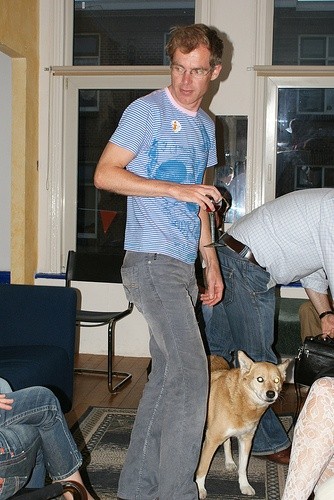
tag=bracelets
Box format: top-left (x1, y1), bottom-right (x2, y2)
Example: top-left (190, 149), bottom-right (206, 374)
top-left (319, 311), bottom-right (334, 319)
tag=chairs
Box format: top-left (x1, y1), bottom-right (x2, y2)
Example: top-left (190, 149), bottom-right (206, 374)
top-left (64, 251), bottom-right (134, 394)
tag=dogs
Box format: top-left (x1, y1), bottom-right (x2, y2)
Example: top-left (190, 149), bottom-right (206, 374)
top-left (194, 350), bottom-right (292, 500)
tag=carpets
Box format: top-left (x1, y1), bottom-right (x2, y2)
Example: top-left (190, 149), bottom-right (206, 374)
top-left (44, 406), bottom-right (295, 500)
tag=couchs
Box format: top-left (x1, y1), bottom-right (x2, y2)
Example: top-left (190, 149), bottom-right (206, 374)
top-left (0, 280), bottom-right (82, 416)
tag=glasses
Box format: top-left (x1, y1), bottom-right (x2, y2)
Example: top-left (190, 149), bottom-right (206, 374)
top-left (170, 62), bottom-right (214, 79)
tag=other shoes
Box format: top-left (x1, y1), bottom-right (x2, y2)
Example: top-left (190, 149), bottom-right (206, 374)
top-left (253, 445), bottom-right (291, 465)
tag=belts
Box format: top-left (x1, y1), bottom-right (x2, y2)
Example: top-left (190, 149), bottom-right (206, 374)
top-left (218, 229), bottom-right (267, 271)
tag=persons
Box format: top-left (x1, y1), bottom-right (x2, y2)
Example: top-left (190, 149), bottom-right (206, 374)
top-left (0, 386), bottom-right (95, 500)
top-left (299, 288), bottom-right (334, 345)
top-left (211, 188), bottom-right (334, 466)
top-left (282, 377), bottom-right (334, 500)
top-left (94, 23), bottom-right (224, 500)
top-left (214, 165), bottom-right (235, 223)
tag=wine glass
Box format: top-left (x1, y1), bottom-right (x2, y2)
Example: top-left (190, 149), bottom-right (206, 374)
top-left (202, 196), bottom-right (227, 248)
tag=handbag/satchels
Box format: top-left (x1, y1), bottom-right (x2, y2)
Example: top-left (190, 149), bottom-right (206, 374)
top-left (293, 333), bottom-right (334, 388)
